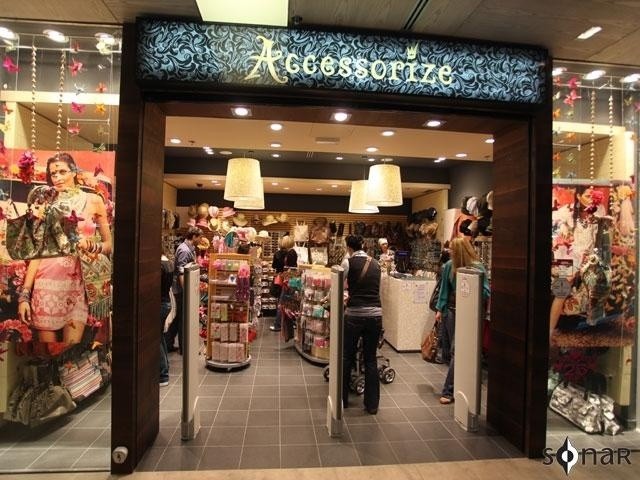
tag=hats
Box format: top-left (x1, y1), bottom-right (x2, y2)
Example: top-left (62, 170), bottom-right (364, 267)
top-left (460, 190), bottom-right (493, 234)
top-left (378, 238), bottom-right (388, 246)
top-left (187, 203), bottom-right (221, 232)
top-left (255, 230), bottom-right (271, 242)
top-left (406, 208), bottom-right (438, 240)
top-left (223, 206), bottom-right (289, 227)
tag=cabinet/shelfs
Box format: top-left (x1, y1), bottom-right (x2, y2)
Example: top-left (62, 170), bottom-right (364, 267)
top-left (205, 255), bottom-right (252, 369)
top-left (293, 265), bottom-right (331, 364)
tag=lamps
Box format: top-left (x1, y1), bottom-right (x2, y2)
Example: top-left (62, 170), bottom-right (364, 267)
top-left (223, 153), bottom-right (261, 201)
top-left (366, 158), bottom-right (404, 207)
top-left (348, 166), bottom-right (380, 214)
top-left (234, 178), bottom-right (265, 210)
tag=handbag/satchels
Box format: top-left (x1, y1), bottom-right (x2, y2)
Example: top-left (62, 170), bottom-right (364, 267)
top-left (3, 350), bottom-right (104, 429)
top-left (6, 185), bottom-right (79, 259)
top-left (293, 225), bottom-right (309, 242)
top-left (311, 247), bottom-right (328, 266)
top-left (311, 226), bottom-right (332, 244)
top-left (421, 321), bottom-right (444, 364)
top-left (429, 279), bottom-right (441, 312)
top-left (293, 247), bottom-right (308, 264)
top-left (77, 236), bottom-right (113, 319)
top-left (547, 367), bottom-right (620, 436)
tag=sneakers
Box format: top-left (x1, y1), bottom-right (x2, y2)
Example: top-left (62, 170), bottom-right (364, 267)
top-left (159, 381), bottom-right (169, 386)
top-left (167, 347), bottom-right (179, 352)
top-left (270, 326), bottom-right (281, 331)
top-left (440, 397), bottom-right (451, 404)
top-left (364, 403), bottom-right (378, 414)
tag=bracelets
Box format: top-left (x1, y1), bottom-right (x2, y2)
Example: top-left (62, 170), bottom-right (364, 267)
top-left (85, 238), bottom-right (102, 255)
top-left (17, 290), bottom-right (31, 304)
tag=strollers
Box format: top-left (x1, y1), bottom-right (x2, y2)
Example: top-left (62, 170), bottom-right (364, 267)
top-left (321, 326), bottom-right (399, 402)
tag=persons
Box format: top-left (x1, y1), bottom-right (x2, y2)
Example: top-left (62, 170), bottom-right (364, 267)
top-left (550, 185), bottom-right (607, 332)
top-left (159, 248), bottom-right (175, 387)
top-left (377, 237), bottom-right (396, 273)
top-left (17, 152), bottom-right (113, 345)
top-left (268, 235), bottom-right (298, 332)
top-left (165, 225), bottom-right (204, 354)
top-left (434, 236), bottom-right (490, 404)
top-left (340, 235), bottom-right (384, 414)
